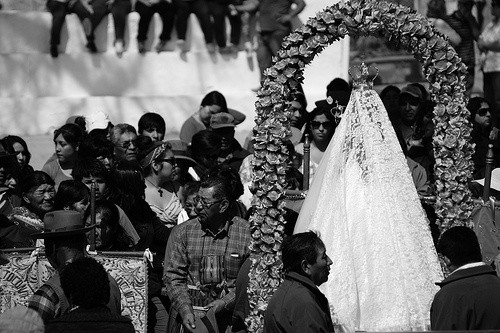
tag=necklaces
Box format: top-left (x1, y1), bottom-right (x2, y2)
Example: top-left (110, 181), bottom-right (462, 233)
top-left (147, 179), bottom-right (163, 197)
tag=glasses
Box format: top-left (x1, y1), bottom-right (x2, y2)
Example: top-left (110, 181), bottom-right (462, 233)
top-left (117, 140), bottom-right (135, 147)
top-left (156, 157), bottom-right (175, 165)
top-left (476, 107), bottom-right (493, 116)
top-left (193, 196), bottom-right (225, 207)
top-left (310, 121), bottom-right (331, 129)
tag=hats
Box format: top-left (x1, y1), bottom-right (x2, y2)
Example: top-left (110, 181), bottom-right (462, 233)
top-left (0, 139), bottom-right (20, 156)
top-left (400, 86), bottom-right (422, 97)
top-left (86, 112), bottom-right (111, 134)
top-left (164, 139), bottom-right (198, 167)
top-left (30, 210), bottom-right (99, 237)
top-left (211, 111), bottom-right (236, 129)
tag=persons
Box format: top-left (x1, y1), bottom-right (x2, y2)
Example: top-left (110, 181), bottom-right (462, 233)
top-left (0, 75), bottom-right (500, 333)
top-left (45, 1), bottom-right (307, 91)
top-left (414, 0), bottom-right (500, 128)
